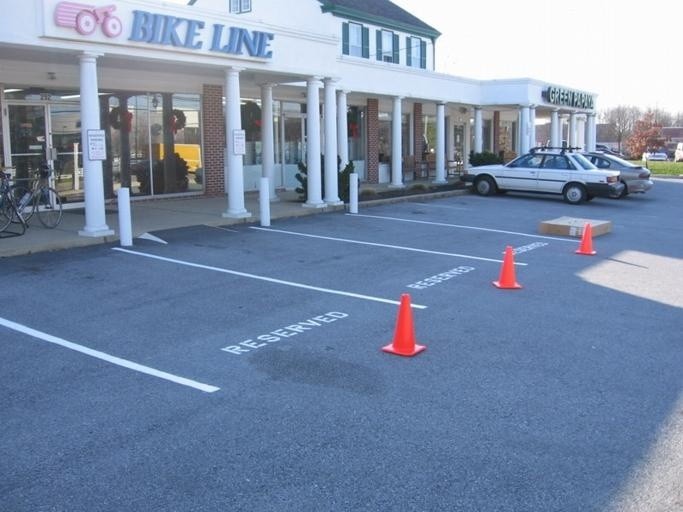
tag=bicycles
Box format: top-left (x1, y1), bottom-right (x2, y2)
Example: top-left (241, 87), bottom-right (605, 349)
top-left (0, 165), bottom-right (62, 233)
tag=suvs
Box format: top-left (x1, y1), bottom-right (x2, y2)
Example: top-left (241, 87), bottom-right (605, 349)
top-left (675, 142), bottom-right (682, 161)
top-left (595, 143), bottom-right (624, 158)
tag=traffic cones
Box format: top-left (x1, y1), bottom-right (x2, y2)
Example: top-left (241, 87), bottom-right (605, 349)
top-left (577, 225), bottom-right (596, 255)
top-left (383, 294), bottom-right (425, 357)
top-left (495, 246), bottom-right (522, 289)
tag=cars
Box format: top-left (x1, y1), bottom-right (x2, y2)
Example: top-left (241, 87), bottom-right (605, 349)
top-left (642, 152), bottom-right (668, 161)
top-left (581, 152), bottom-right (653, 199)
top-left (460, 151), bottom-right (620, 205)
